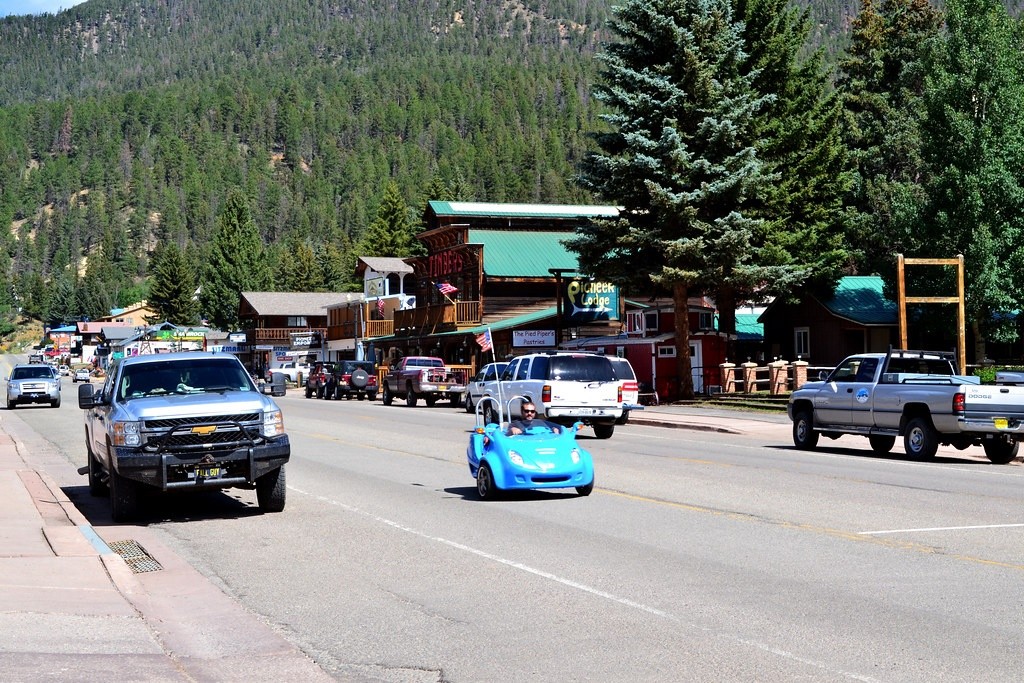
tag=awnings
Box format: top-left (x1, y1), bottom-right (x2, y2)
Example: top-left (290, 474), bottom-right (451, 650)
top-left (115, 333), bottom-right (145, 346)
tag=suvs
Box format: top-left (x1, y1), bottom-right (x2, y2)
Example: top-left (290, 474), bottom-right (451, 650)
top-left (305, 363), bottom-right (336, 399)
top-left (324, 360), bottom-right (379, 401)
top-left (3, 364), bottom-right (61, 409)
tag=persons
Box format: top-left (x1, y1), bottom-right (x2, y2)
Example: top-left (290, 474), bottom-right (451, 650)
top-left (508, 402), bottom-right (559, 436)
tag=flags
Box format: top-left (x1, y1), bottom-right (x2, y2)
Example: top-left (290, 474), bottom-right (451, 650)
top-left (476, 330), bottom-right (492, 352)
top-left (434, 283), bottom-right (457, 295)
top-left (376, 296), bottom-right (384, 312)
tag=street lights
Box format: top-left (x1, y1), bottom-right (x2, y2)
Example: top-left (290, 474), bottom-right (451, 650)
top-left (176, 328), bottom-right (188, 352)
top-left (345, 292), bottom-right (366, 361)
top-left (56, 333), bottom-right (61, 367)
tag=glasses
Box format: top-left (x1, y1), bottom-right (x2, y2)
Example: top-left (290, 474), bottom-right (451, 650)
top-left (523, 409), bottom-right (535, 413)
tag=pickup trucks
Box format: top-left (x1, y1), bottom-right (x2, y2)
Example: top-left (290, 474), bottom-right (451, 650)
top-left (383, 356), bottom-right (466, 408)
top-left (72, 368), bottom-right (90, 384)
top-left (27, 354), bottom-right (41, 365)
top-left (268, 362), bottom-right (313, 382)
top-left (786, 344), bottom-right (1024, 464)
top-left (77, 352), bottom-right (292, 522)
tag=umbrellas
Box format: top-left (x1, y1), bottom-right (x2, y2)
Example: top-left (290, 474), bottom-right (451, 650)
top-left (355, 340), bottom-right (365, 361)
top-left (366, 340), bottom-right (376, 365)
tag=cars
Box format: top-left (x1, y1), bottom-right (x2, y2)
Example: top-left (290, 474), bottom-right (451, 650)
top-left (47, 362), bottom-right (60, 374)
top-left (59, 365), bottom-right (69, 376)
top-left (481, 350), bottom-right (623, 439)
top-left (602, 354), bottom-right (645, 426)
top-left (466, 419), bottom-right (596, 500)
top-left (465, 362), bottom-right (511, 413)
